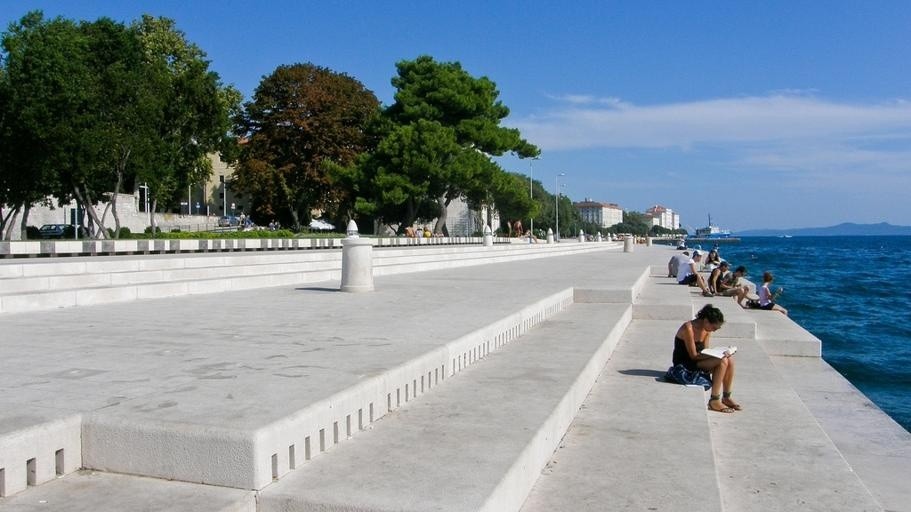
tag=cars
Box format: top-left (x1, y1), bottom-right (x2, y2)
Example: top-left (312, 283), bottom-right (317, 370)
top-left (216, 214), bottom-right (240, 226)
top-left (37, 223), bottom-right (70, 238)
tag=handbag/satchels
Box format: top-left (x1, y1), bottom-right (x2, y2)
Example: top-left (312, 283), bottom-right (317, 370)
top-left (666, 364), bottom-right (713, 391)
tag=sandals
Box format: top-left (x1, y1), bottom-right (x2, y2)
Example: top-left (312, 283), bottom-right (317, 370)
top-left (708, 392), bottom-right (743, 413)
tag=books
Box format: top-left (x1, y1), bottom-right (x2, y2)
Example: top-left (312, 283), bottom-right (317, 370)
top-left (703, 346), bottom-right (737, 359)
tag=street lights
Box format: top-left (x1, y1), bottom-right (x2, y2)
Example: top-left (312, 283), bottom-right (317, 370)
top-left (231, 202), bottom-right (236, 217)
top-left (219, 173), bottom-right (227, 215)
top-left (529, 156), bottom-right (542, 243)
top-left (187, 182), bottom-right (197, 215)
top-left (559, 182), bottom-right (567, 194)
top-left (554, 171), bottom-right (566, 241)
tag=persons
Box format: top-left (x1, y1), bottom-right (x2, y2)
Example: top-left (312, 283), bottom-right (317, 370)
top-left (423, 227), bottom-right (432, 237)
top-left (514, 220), bottom-right (523, 238)
top-left (667, 248), bottom-right (787, 316)
top-left (268, 218), bottom-right (275, 231)
top-left (240, 211), bottom-right (245, 225)
top-left (245, 215), bottom-right (254, 227)
top-left (275, 220), bottom-right (281, 230)
top-left (506, 218), bottom-right (512, 237)
top-left (415, 227), bottom-right (423, 238)
top-left (672, 303), bottom-right (743, 413)
top-left (404, 225), bottom-right (415, 238)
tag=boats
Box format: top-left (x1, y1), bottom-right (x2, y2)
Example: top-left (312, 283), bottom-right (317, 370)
top-left (688, 211), bottom-right (736, 238)
top-left (781, 234), bottom-right (792, 238)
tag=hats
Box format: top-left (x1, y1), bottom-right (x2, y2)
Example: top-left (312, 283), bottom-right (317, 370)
top-left (693, 250), bottom-right (704, 256)
